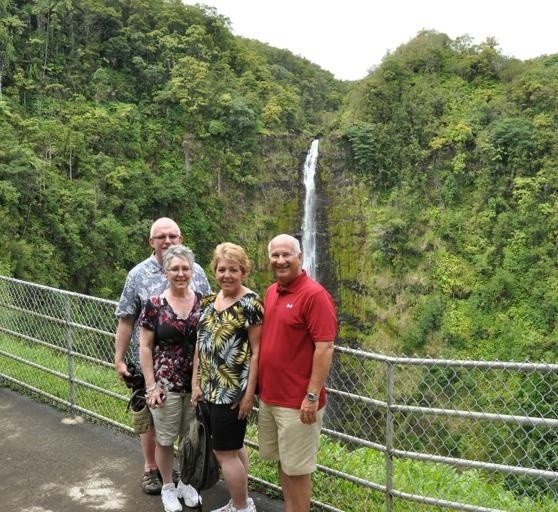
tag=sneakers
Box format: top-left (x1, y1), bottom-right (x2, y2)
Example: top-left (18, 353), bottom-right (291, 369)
top-left (142, 467), bottom-right (182, 495)
top-left (210, 498), bottom-right (257, 512)
top-left (176, 478), bottom-right (202, 508)
top-left (161, 482), bottom-right (182, 512)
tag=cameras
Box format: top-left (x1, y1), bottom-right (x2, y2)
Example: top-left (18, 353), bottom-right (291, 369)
top-left (123, 363), bottom-right (146, 389)
top-left (155, 394), bottom-right (167, 408)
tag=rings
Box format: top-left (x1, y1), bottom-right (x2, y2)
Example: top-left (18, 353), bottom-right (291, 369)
top-left (190, 399), bottom-right (192, 402)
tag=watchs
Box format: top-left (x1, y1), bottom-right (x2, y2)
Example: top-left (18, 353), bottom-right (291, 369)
top-left (306, 392), bottom-right (320, 401)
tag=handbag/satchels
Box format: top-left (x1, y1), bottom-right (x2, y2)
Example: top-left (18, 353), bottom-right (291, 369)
top-left (178, 415), bottom-right (219, 490)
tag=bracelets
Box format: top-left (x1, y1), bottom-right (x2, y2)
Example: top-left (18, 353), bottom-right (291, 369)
top-left (146, 383), bottom-right (157, 392)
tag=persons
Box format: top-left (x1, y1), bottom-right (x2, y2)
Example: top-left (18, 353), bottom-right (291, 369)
top-left (257, 233), bottom-right (337, 512)
top-left (191, 242), bottom-right (264, 512)
top-left (113, 217), bottom-right (211, 495)
top-left (137, 244), bottom-right (213, 512)
